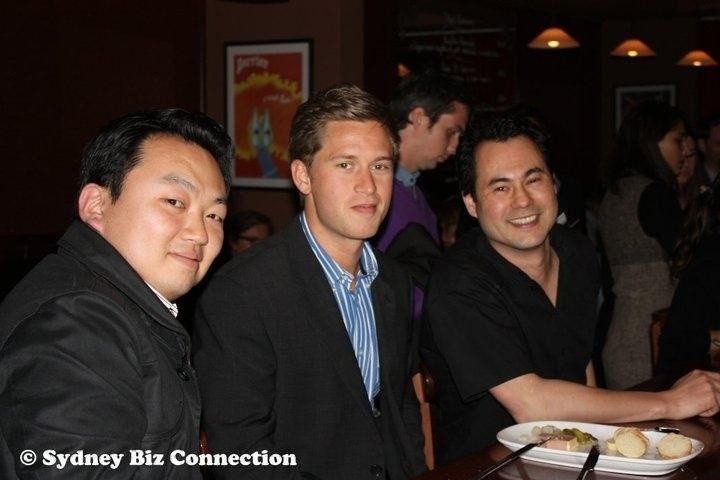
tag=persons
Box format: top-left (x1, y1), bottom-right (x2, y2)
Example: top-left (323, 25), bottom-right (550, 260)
top-left (419, 118), bottom-right (720, 479)
top-left (225, 209), bottom-right (274, 261)
top-left (695, 118), bottom-right (720, 199)
top-left (596, 110), bottom-right (720, 387)
top-left (187, 85), bottom-right (429, 480)
top-left (662, 132), bottom-right (699, 204)
top-left (0, 107), bottom-right (236, 480)
top-left (374, 79), bottom-right (470, 321)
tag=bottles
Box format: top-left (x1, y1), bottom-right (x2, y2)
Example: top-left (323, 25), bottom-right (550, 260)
top-left (261, 110), bottom-right (271, 149)
top-left (251, 108), bottom-right (261, 149)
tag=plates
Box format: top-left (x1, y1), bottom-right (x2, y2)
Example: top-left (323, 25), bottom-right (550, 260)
top-left (497, 421), bottom-right (704, 475)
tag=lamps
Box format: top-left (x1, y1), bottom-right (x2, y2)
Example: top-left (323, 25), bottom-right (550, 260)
top-left (528, 26), bottom-right (581, 50)
top-left (677, 48), bottom-right (717, 69)
top-left (611, 39), bottom-right (660, 59)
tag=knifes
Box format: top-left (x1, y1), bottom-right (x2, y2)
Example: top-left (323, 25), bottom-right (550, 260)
top-left (576, 443), bottom-right (602, 480)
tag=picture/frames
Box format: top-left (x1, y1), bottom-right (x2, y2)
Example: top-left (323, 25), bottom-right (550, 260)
top-left (225, 39), bottom-right (314, 193)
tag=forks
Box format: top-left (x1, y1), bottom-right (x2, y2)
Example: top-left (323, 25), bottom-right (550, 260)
top-left (477, 433), bottom-right (555, 480)
